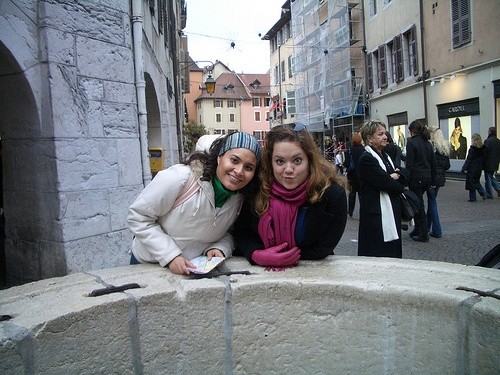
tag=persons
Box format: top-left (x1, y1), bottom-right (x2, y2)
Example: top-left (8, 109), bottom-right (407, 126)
top-left (191, 133), bottom-right (200, 144)
top-left (448, 117), bottom-right (467, 159)
top-left (127, 131), bottom-right (262, 277)
top-left (396, 126), bottom-right (406, 153)
top-left (316, 117), bottom-right (500, 257)
top-left (232, 123), bottom-right (347, 271)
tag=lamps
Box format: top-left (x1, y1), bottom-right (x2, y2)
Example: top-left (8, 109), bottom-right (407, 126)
top-left (178, 61), bottom-right (217, 97)
top-left (451, 73), bottom-right (467, 80)
top-left (429, 80), bottom-right (439, 86)
top-left (441, 77), bottom-right (451, 83)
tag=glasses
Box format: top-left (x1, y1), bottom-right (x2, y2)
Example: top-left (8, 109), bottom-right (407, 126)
top-left (270, 122), bottom-right (309, 133)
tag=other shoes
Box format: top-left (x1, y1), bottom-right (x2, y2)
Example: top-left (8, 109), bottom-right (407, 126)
top-left (482, 192), bottom-right (487, 200)
top-left (497, 188), bottom-right (500, 197)
top-left (428, 232), bottom-right (443, 237)
top-left (468, 199), bottom-right (477, 202)
top-left (412, 234), bottom-right (430, 242)
top-left (487, 196), bottom-right (493, 199)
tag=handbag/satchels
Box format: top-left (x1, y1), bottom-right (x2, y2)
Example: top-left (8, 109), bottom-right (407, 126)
top-left (397, 190), bottom-right (421, 221)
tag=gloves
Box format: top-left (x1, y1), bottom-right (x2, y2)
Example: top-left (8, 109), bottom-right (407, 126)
top-left (252, 242), bottom-right (301, 267)
